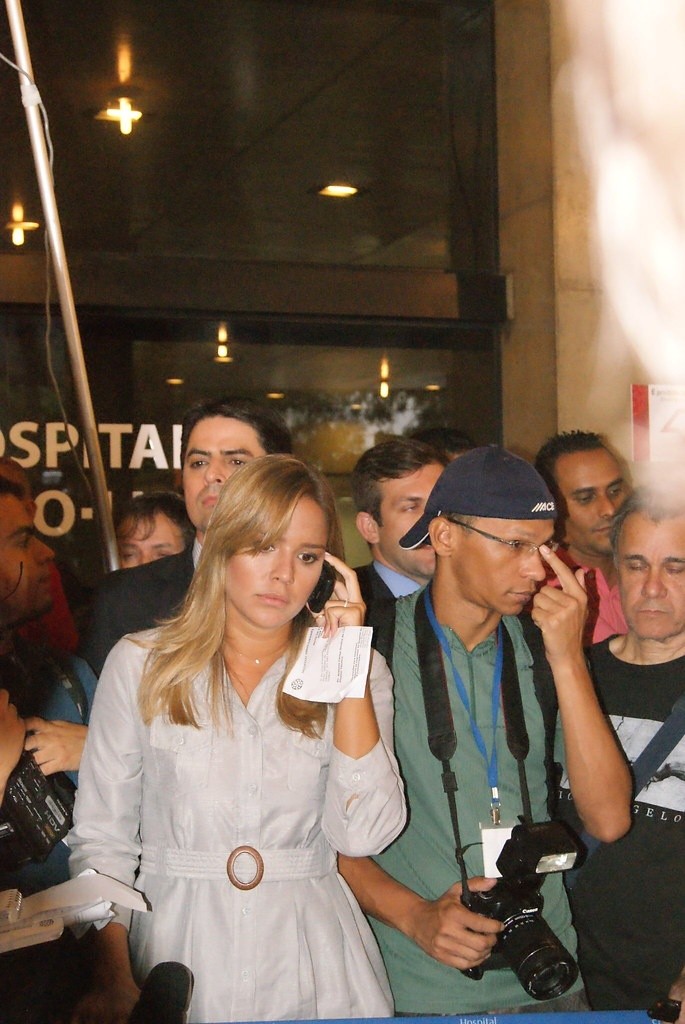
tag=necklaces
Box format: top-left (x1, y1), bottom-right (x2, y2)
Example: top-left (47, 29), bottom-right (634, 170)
top-left (232, 648), bottom-right (269, 664)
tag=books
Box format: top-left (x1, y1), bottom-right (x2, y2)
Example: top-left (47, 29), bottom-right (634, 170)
top-left (0, 889), bottom-right (22, 924)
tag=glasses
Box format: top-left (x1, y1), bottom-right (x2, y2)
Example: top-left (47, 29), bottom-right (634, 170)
top-left (444, 515), bottom-right (559, 563)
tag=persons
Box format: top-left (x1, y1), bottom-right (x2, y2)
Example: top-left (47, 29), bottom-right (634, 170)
top-left (412, 427), bottom-right (477, 462)
top-left (340, 438), bottom-right (454, 670)
top-left (546, 480), bottom-right (685, 1024)
top-left (87, 396), bottom-right (295, 677)
top-left (65, 454), bottom-right (410, 1024)
top-left (524, 431), bottom-right (630, 647)
top-left (111, 491), bottom-right (196, 570)
top-left (336, 444), bottom-right (634, 1013)
top-left (0, 458), bottom-right (97, 1024)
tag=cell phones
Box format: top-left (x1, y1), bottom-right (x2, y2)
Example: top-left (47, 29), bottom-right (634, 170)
top-left (307, 561), bottom-right (335, 612)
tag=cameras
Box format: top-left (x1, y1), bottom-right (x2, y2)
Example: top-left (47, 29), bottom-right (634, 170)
top-left (459, 821), bottom-right (587, 1000)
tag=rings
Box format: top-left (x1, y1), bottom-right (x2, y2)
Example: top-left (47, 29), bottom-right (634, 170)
top-left (344, 600), bottom-right (349, 608)
top-left (315, 614), bottom-right (325, 620)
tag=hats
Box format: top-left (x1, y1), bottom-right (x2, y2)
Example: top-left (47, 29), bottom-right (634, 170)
top-left (397, 443), bottom-right (559, 550)
